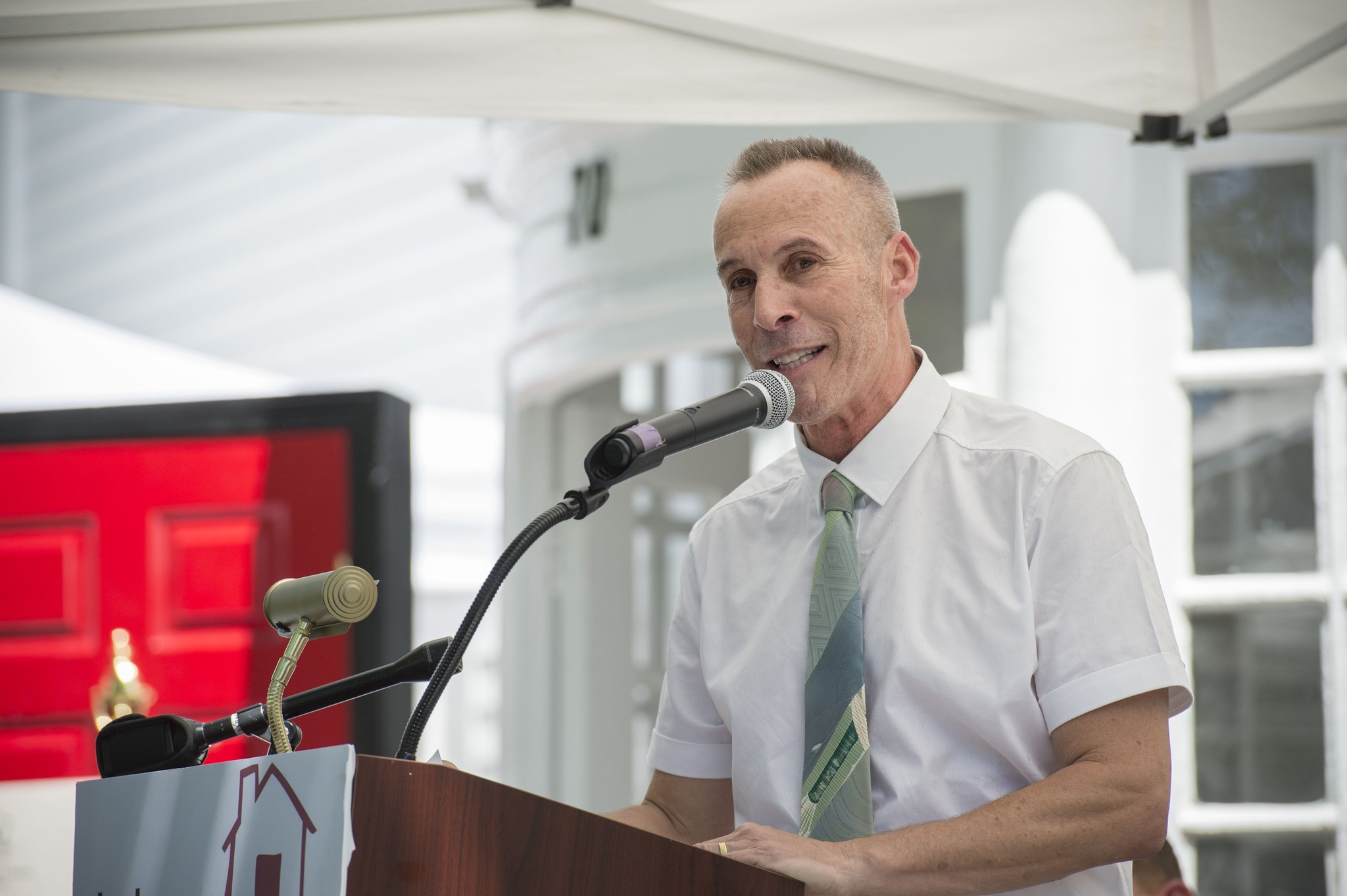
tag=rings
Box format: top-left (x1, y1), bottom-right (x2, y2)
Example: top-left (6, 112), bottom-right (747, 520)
top-left (716, 840), bottom-right (727, 856)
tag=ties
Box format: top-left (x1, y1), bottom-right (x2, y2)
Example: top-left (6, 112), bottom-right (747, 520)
top-left (799, 468), bottom-right (874, 844)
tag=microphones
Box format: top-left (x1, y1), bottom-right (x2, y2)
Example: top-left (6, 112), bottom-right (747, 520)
top-left (95, 637), bottom-right (464, 779)
top-left (603, 369), bottom-right (795, 473)
top-left (264, 566), bottom-right (378, 754)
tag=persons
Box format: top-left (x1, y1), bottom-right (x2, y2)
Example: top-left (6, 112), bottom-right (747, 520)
top-left (604, 135), bottom-right (1192, 896)
top-left (1133, 842), bottom-right (1198, 896)
